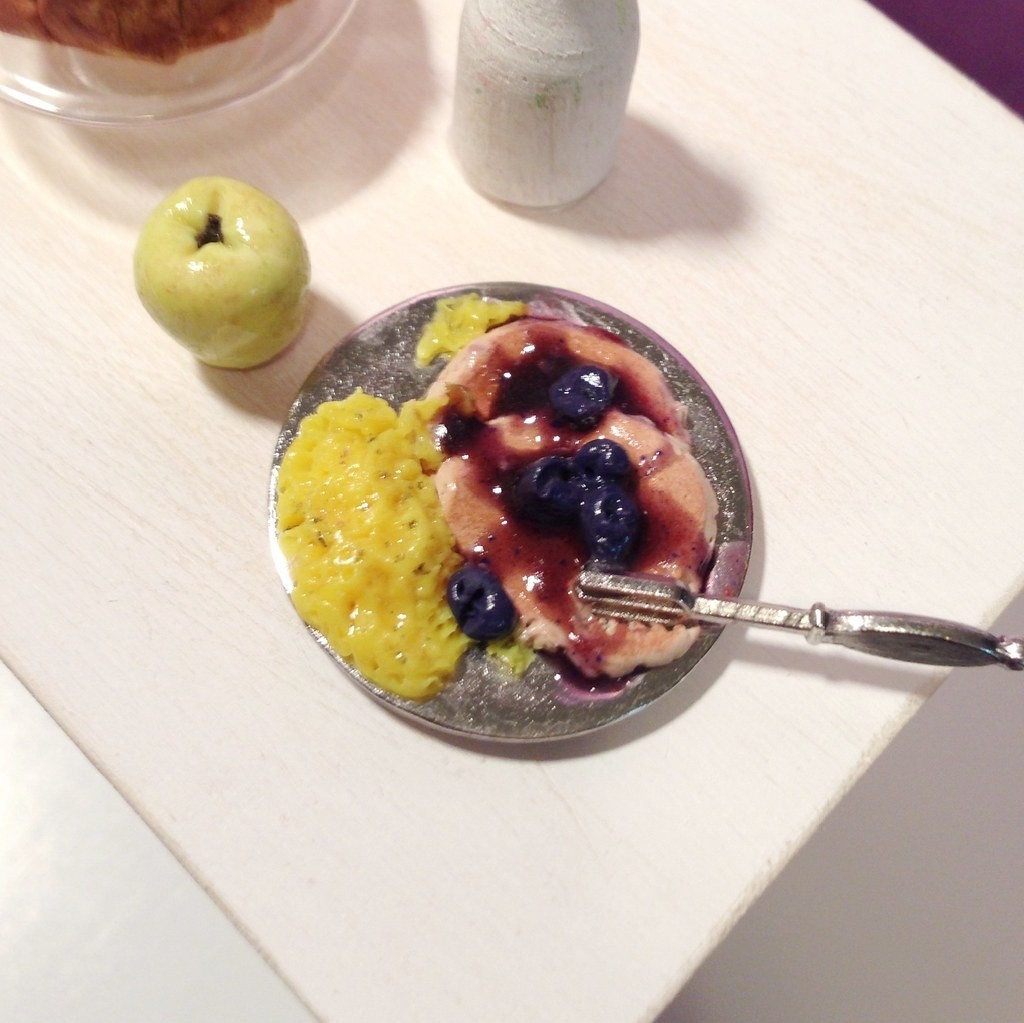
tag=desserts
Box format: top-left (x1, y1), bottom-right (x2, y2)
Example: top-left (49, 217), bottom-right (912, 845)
top-left (264, 282), bottom-right (755, 745)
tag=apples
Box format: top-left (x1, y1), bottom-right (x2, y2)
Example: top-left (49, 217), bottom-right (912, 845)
top-left (132, 175), bottom-right (310, 369)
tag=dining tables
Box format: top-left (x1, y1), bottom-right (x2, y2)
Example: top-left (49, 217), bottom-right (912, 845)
top-left (0, 0), bottom-right (1024, 1023)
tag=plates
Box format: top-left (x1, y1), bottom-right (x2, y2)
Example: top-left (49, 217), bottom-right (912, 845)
top-left (0, 0), bottom-right (355, 126)
top-left (267, 281), bottom-right (754, 744)
